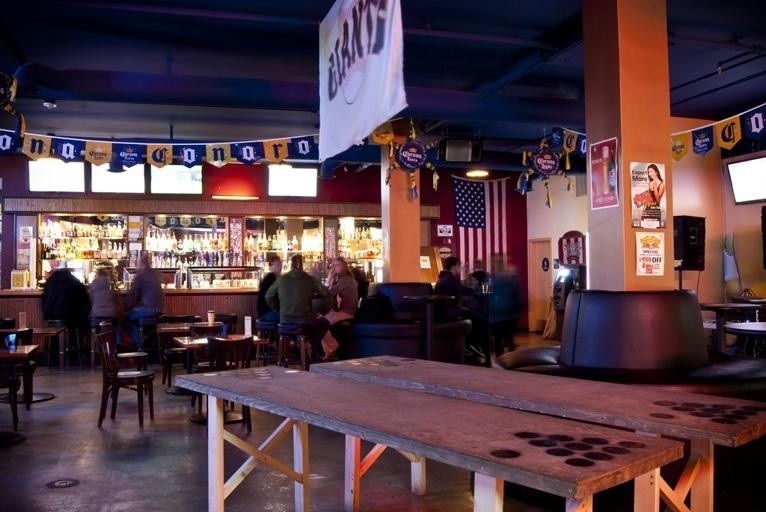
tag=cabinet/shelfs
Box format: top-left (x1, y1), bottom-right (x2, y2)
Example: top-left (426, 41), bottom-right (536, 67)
top-left (13, 210), bottom-right (386, 285)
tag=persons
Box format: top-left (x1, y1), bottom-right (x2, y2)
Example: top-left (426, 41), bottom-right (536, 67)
top-left (633, 164), bottom-right (665, 229)
top-left (257, 256), bottom-right (281, 321)
top-left (316, 257), bottom-right (359, 361)
top-left (88, 271), bottom-right (123, 353)
top-left (125, 253), bottom-right (162, 352)
top-left (432, 257), bottom-right (482, 321)
top-left (264, 256), bottom-right (332, 363)
top-left (42, 262), bottom-right (91, 335)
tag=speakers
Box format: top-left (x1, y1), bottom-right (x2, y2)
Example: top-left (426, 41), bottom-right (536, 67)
top-left (673, 216), bottom-right (706, 271)
top-left (439, 139), bottom-right (481, 163)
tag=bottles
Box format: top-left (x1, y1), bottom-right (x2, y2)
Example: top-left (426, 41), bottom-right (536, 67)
top-left (257, 233), bottom-right (262, 248)
top-left (171, 231), bottom-right (177, 250)
top-left (217, 248), bottom-right (223, 267)
top-left (182, 258), bottom-right (189, 287)
top-left (146, 226), bottom-right (171, 251)
top-left (244, 231), bottom-right (248, 250)
top-left (288, 239), bottom-right (292, 250)
top-left (189, 250), bottom-right (218, 266)
top-left (176, 257), bottom-right (183, 273)
top-left (47, 222), bottom-right (125, 238)
top-left (194, 234), bottom-right (199, 249)
top-left (165, 253), bottom-right (171, 269)
top-left (262, 233), bottom-right (267, 248)
top-left (129, 250), bottom-right (137, 269)
top-left (188, 234), bottom-right (193, 250)
top-left (244, 250), bottom-right (268, 269)
top-left (208, 233), bottom-right (213, 249)
top-left (183, 233), bottom-right (189, 250)
top-left (248, 232), bottom-right (255, 250)
top-left (267, 235), bottom-right (271, 249)
top-left (293, 235), bottom-right (298, 251)
top-left (153, 252), bottom-right (165, 269)
top-left (223, 248), bottom-right (229, 267)
top-left (199, 234), bottom-right (204, 250)
top-left (41, 241), bottom-right (128, 258)
top-left (302, 254), bottom-right (325, 274)
top-left (218, 234), bottom-right (223, 250)
top-left (172, 252), bottom-right (176, 269)
top-left (353, 225), bottom-right (371, 241)
top-left (272, 235), bottom-right (277, 249)
top-left (224, 233), bottom-right (229, 249)
top-left (204, 231), bottom-right (209, 249)
top-left (213, 232), bottom-right (218, 249)
top-left (229, 248), bottom-right (233, 266)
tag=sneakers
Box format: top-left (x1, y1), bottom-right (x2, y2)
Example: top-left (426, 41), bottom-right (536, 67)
top-left (466, 343), bottom-right (486, 356)
top-left (323, 343), bottom-right (339, 360)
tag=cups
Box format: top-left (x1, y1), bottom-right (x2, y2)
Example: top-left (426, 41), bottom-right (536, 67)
top-left (208, 310), bottom-right (215, 326)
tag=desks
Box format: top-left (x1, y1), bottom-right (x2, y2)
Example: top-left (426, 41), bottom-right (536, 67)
top-left (174, 333), bottom-right (252, 396)
top-left (403, 293), bottom-right (455, 362)
top-left (698, 303), bottom-right (761, 359)
top-left (313, 354), bottom-right (766, 512)
top-left (2, 344), bottom-right (39, 430)
top-left (171, 365), bottom-right (684, 512)
top-left (2, 284), bottom-right (255, 362)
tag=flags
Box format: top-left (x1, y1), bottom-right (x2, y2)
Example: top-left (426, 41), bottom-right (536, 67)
top-left (452, 176), bottom-right (508, 280)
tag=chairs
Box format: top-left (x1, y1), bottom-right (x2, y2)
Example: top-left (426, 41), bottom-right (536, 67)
top-left (94, 332), bottom-right (154, 426)
top-left (99, 319), bottom-right (148, 392)
top-left (194, 337), bottom-right (255, 430)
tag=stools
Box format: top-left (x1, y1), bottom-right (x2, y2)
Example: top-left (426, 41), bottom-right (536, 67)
top-left (46, 320), bottom-right (74, 366)
top-left (184, 313), bottom-right (203, 322)
top-left (8, 359), bottom-right (36, 409)
top-left (215, 312), bottom-right (237, 333)
top-left (254, 320), bottom-right (279, 369)
top-left (0, 373), bottom-right (21, 433)
top-left (164, 347), bottom-right (193, 381)
top-left (278, 324), bottom-right (309, 372)
top-left (0, 317), bottom-right (15, 329)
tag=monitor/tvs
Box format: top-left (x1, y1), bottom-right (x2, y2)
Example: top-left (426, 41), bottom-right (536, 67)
top-left (27, 157), bottom-right (88, 195)
top-left (723, 151), bottom-right (766, 209)
top-left (89, 161), bottom-right (148, 196)
top-left (149, 163), bottom-right (205, 198)
top-left (267, 163), bottom-right (319, 198)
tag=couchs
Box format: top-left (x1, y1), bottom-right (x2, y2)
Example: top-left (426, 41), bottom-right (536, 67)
top-left (355, 280), bottom-right (472, 361)
top-left (472, 287), bottom-right (766, 512)
